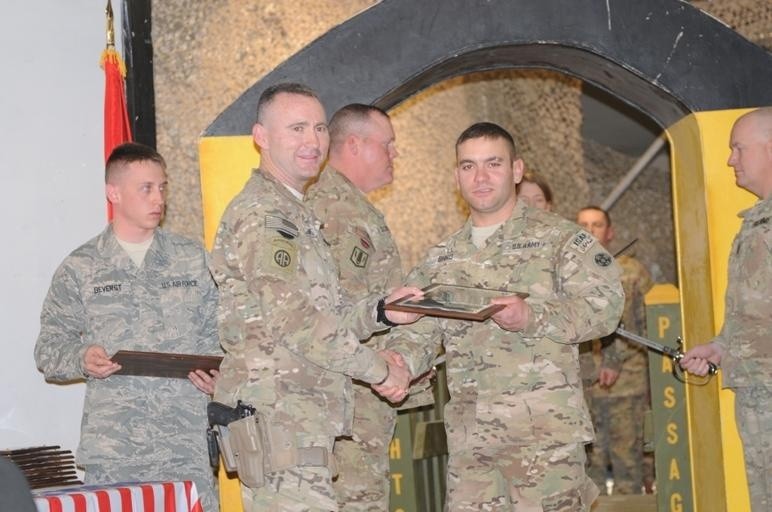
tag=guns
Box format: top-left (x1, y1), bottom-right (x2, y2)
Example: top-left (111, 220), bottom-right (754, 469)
top-left (207, 398), bottom-right (256, 429)
